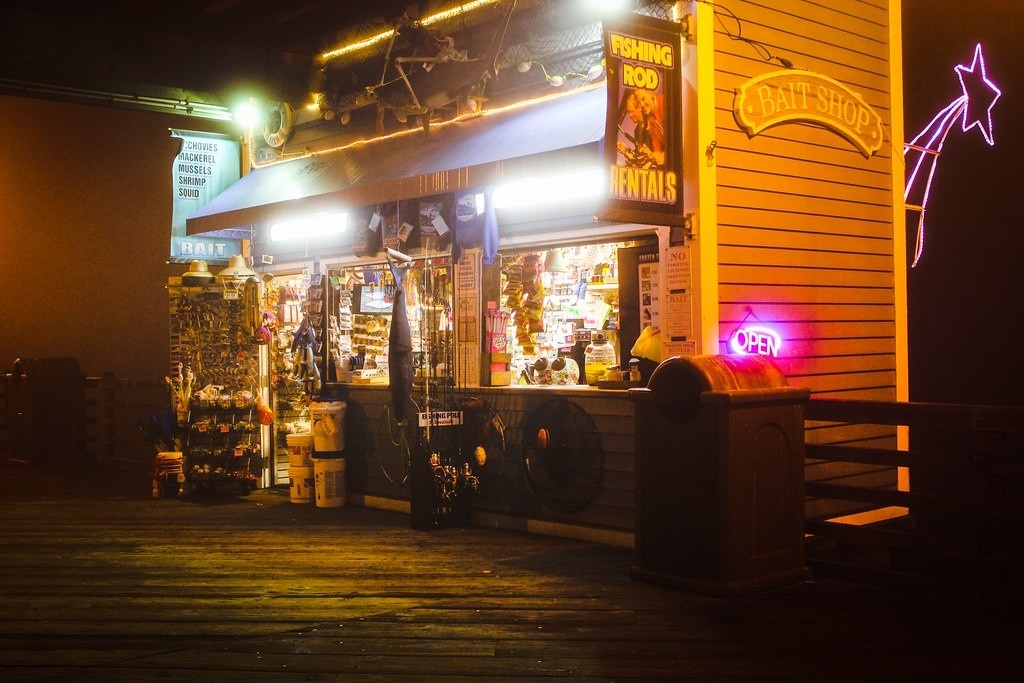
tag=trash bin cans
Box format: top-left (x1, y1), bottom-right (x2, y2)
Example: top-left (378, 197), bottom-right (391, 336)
top-left (627, 354), bottom-right (806, 596)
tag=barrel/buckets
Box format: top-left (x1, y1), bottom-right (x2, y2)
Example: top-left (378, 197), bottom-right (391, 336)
top-left (286, 434), bottom-right (314, 466)
top-left (288, 467), bottom-right (312, 503)
top-left (309, 456), bottom-right (347, 507)
top-left (309, 401), bottom-right (347, 452)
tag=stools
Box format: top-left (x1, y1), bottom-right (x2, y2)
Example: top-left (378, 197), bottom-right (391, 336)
top-left (152, 452), bottom-right (184, 498)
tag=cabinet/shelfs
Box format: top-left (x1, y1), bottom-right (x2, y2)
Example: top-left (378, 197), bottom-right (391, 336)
top-left (179, 384), bottom-right (261, 497)
